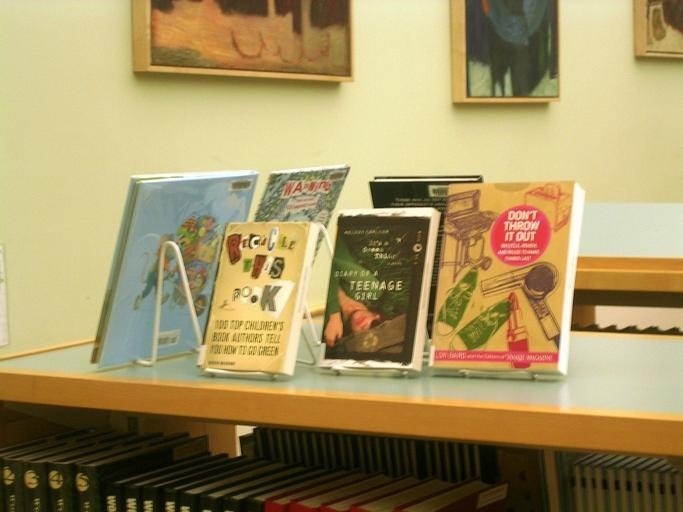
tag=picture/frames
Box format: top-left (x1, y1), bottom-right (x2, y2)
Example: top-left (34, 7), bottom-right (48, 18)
top-left (450, 0), bottom-right (560, 104)
top-left (132, 0), bottom-right (354, 83)
top-left (634, 0), bottom-right (682, 59)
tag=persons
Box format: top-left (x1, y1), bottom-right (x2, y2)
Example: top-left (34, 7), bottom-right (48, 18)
top-left (1, 424), bottom-right (683, 512)
top-left (323, 237), bottom-right (385, 351)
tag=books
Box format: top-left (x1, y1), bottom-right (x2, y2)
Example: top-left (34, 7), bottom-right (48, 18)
top-left (86, 167), bottom-right (259, 374)
top-left (255, 165), bottom-right (350, 269)
top-left (426, 181), bottom-right (585, 375)
top-left (367, 176), bottom-right (483, 341)
top-left (311, 208), bottom-right (442, 373)
top-left (196, 219), bottom-right (318, 377)
